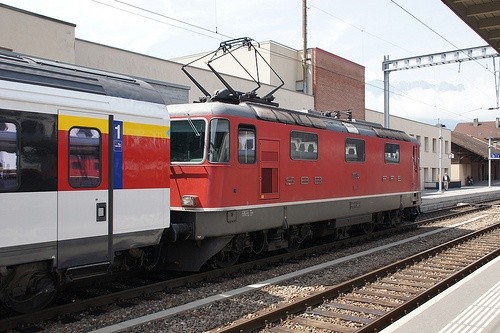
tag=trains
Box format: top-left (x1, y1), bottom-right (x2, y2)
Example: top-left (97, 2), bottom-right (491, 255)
top-left (0, 37), bottom-right (423, 329)
